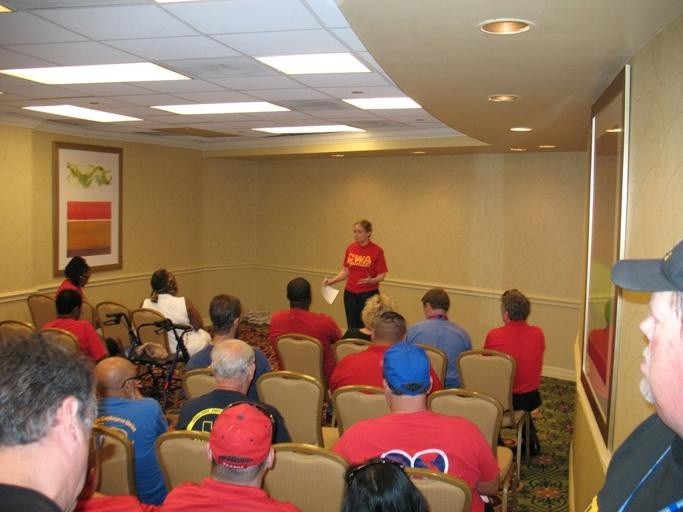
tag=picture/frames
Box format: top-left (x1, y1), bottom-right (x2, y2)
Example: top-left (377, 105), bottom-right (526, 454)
top-left (580, 63), bottom-right (631, 452)
top-left (51, 140), bottom-right (123, 279)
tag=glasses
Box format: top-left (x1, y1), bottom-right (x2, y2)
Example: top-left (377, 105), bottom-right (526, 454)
top-left (345, 458), bottom-right (404, 485)
top-left (120, 375), bottom-right (142, 388)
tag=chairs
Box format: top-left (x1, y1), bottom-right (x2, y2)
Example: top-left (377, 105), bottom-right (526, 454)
top-left (333, 338), bottom-right (372, 364)
top-left (415, 343), bottom-right (446, 388)
top-left (1, 319), bottom-right (36, 346)
top-left (93, 423), bottom-right (135, 499)
top-left (154, 430), bottom-right (214, 492)
top-left (332, 385), bottom-right (391, 438)
top-left (77, 300), bottom-right (190, 410)
top-left (457, 348), bottom-right (529, 478)
top-left (262, 442), bottom-right (349, 511)
top-left (273, 333), bottom-right (337, 426)
top-left (27, 293), bottom-right (58, 327)
top-left (256, 371), bottom-right (338, 450)
top-left (38, 328), bottom-right (79, 358)
top-left (427, 388), bottom-right (518, 511)
top-left (182, 368), bottom-right (217, 401)
top-left (403, 465), bottom-right (472, 511)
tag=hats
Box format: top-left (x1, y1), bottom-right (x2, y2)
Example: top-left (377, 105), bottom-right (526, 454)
top-left (610, 238), bottom-right (682, 292)
top-left (209, 403), bottom-right (272, 469)
top-left (382, 340), bottom-right (430, 396)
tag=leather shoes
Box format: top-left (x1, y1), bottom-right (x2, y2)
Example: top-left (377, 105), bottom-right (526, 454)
top-left (510, 440), bottom-right (540, 452)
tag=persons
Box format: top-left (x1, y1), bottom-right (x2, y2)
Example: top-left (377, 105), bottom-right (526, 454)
top-left (320, 219), bottom-right (388, 345)
top-left (485, 289), bottom-right (545, 453)
top-left (140, 270), bottom-right (211, 356)
top-left (341, 457), bottom-right (429, 511)
top-left (75, 403), bottom-right (301, 512)
top-left (0, 320), bottom-right (100, 512)
top-left (41, 289), bottom-right (108, 363)
top-left (177, 292), bottom-right (292, 444)
top-left (335, 344), bottom-right (501, 511)
top-left (95, 356), bottom-right (172, 505)
top-left (56, 256), bottom-right (91, 301)
top-left (267, 280), bottom-right (342, 422)
top-left (331, 287), bottom-right (471, 394)
top-left (587, 297), bottom-right (611, 410)
top-left (579, 240), bottom-right (683, 512)
